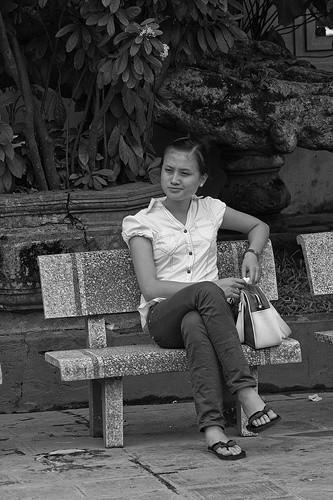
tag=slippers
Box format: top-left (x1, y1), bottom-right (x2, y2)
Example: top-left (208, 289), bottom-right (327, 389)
top-left (207, 440), bottom-right (246, 459)
top-left (246, 406), bottom-right (280, 433)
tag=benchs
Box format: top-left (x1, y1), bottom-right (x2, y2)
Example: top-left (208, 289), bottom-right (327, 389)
top-left (38, 239), bottom-right (302, 449)
top-left (297, 232), bottom-right (333, 344)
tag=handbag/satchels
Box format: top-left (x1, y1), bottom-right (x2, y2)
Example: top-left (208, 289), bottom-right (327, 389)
top-left (226, 283), bottom-right (292, 349)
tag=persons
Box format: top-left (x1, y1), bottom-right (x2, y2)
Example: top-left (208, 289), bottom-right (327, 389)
top-left (122, 137), bottom-right (281, 461)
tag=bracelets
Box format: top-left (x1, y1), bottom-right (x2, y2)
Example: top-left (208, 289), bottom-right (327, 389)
top-left (243, 249), bottom-right (260, 261)
